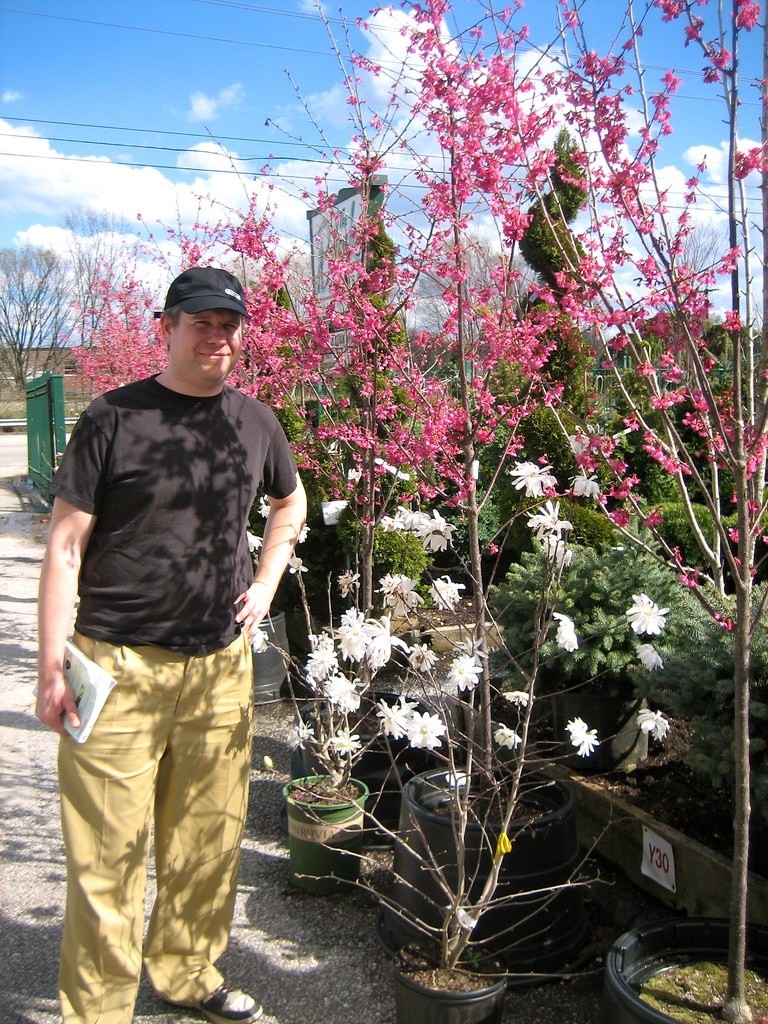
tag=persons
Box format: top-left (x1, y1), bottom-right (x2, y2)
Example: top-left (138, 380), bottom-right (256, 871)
top-left (36, 266), bottom-right (307, 1024)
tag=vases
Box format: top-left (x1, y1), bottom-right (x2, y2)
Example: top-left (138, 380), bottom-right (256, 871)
top-left (549, 690), bottom-right (648, 769)
top-left (288, 695), bottom-right (428, 846)
top-left (283, 776), bottom-right (370, 896)
top-left (386, 767), bottom-right (582, 976)
top-left (251, 609), bottom-right (289, 706)
top-left (390, 941), bottom-right (509, 1024)
top-left (600, 918), bottom-right (768, 1024)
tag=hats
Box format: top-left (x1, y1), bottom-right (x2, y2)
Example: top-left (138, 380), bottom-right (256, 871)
top-left (154, 266), bottom-right (251, 320)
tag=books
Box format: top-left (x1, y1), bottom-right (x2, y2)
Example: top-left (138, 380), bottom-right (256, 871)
top-left (32, 640), bottom-right (118, 743)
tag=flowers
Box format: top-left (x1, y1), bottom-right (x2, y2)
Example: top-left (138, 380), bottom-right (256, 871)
top-left (70, 0), bottom-right (768, 1015)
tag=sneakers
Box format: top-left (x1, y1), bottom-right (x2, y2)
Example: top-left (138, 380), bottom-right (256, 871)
top-left (195, 984), bottom-right (263, 1024)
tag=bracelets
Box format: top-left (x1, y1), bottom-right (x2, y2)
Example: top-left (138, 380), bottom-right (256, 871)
top-left (253, 577), bottom-right (277, 593)
